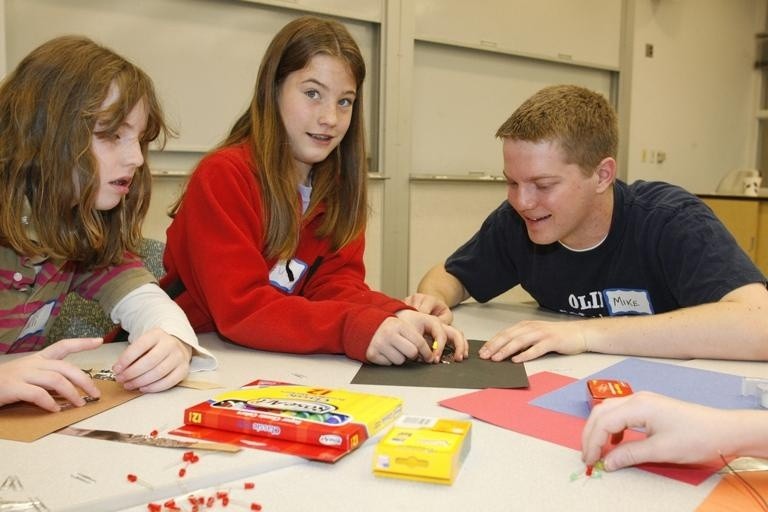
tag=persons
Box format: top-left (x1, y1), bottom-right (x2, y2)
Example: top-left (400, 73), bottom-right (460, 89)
top-left (402, 84), bottom-right (768, 364)
top-left (582, 390), bottom-right (768, 471)
top-left (160, 15), bottom-right (469, 366)
top-left (0, 36), bottom-right (217, 411)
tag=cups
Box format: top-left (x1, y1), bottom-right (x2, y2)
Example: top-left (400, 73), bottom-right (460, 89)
top-left (743, 176), bottom-right (762, 197)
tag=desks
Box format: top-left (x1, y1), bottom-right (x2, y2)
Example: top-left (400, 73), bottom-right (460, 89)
top-left (2, 296), bottom-right (765, 507)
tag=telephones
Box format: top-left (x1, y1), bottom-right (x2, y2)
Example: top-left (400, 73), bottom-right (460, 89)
top-left (719, 167), bottom-right (759, 194)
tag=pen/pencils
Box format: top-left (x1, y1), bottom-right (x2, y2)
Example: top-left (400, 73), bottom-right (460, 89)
top-left (220, 399), bottom-right (348, 425)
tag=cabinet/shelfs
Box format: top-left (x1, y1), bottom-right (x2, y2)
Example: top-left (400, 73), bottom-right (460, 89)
top-left (697, 196), bottom-right (766, 276)
